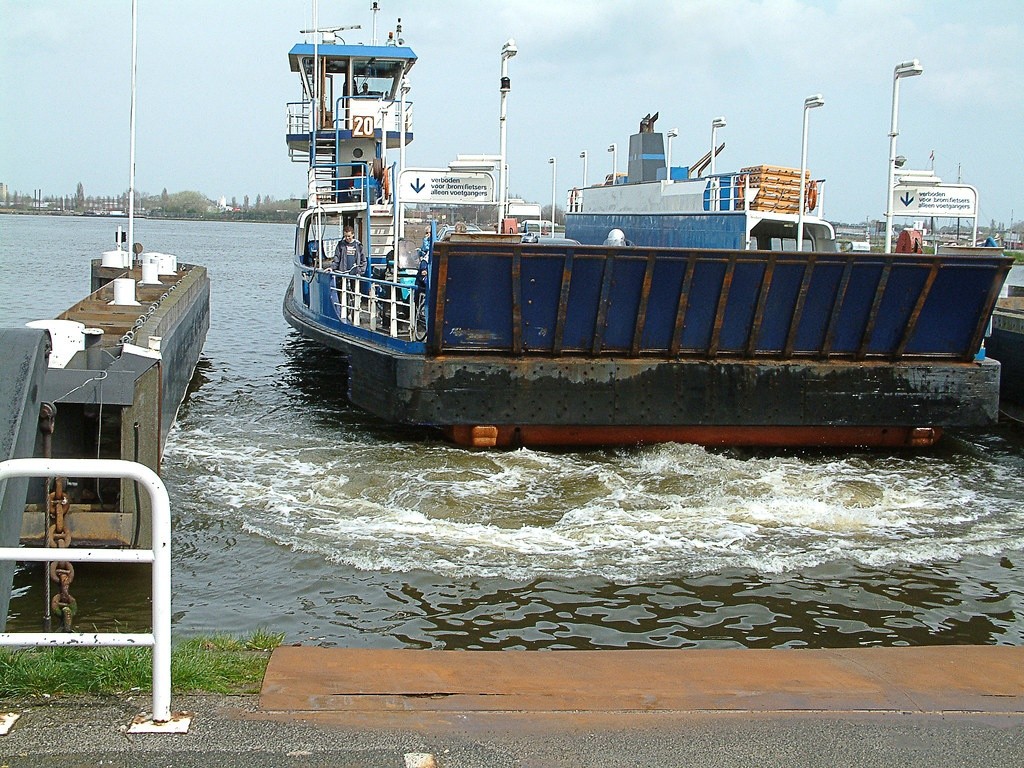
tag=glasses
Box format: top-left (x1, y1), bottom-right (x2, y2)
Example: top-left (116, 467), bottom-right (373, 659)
top-left (426, 232), bottom-right (430, 234)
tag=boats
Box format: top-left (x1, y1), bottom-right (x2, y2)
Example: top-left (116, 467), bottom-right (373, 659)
top-left (281, 3), bottom-right (1019, 456)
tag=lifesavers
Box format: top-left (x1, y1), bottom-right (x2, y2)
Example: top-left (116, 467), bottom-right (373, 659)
top-left (808, 180), bottom-right (817, 211)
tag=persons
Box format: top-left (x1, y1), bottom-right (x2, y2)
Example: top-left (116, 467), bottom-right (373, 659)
top-left (360, 83), bottom-right (374, 99)
top-left (343, 78), bottom-right (358, 129)
top-left (387, 237), bottom-right (406, 276)
top-left (455, 222), bottom-right (466, 233)
top-left (324, 225), bottom-right (367, 323)
top-left (420, 225), bottom-right (431, 256)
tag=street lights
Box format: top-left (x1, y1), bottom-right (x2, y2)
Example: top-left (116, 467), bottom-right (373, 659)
top-left (885, 58), bottom-right (924, 250)
top-left (475, 210), bottom-right (480, 227)
top-left (710, 115), bottom-right (727, 174)
top-left (795, 93), bottom-right (825, 251)
top-left (667, 127), bottom-right (678, 181)
top-left (607, 143), bottom-right (617, 185)
top-left (497, 37), bottom-right (517, 234)
top-left (547, 157), bottom-right (556, 238)
top-left (580, 150), bottom-right (587, 187)
